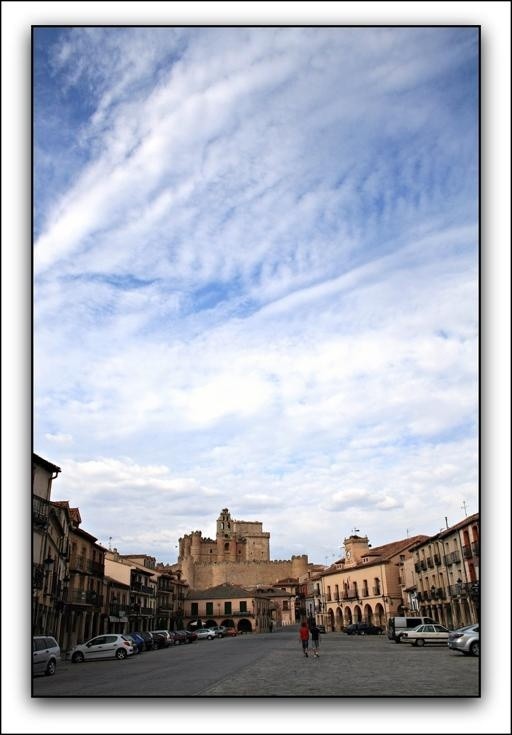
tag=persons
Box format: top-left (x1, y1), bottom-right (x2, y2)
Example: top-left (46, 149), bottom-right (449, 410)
top-left (298, 622), bottom-right (308, 658)
top-left (310, 623), bottom-right (320, 658)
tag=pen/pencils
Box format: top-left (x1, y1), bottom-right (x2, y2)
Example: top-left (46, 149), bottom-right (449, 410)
top-left (387, 616), bottom-right (441, 644)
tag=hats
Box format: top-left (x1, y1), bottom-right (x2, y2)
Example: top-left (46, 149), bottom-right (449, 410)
top-left (342, 622), bottom-right (384, 636)
top-left (65, 625), bottom-right (238, 664)
top-left (447, 623), bottom-right (480, 657)
top-left (400, 623), bottom-right (455, 647)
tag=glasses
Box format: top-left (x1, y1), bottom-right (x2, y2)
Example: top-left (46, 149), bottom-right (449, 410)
top-left (456, 577), bottom-right (467, 590)
top-left (40, 554), bottom-right (54, 579)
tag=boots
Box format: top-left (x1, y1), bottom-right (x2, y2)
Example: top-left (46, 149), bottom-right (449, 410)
top-left (32, 634), bottom-right (62, 677)
